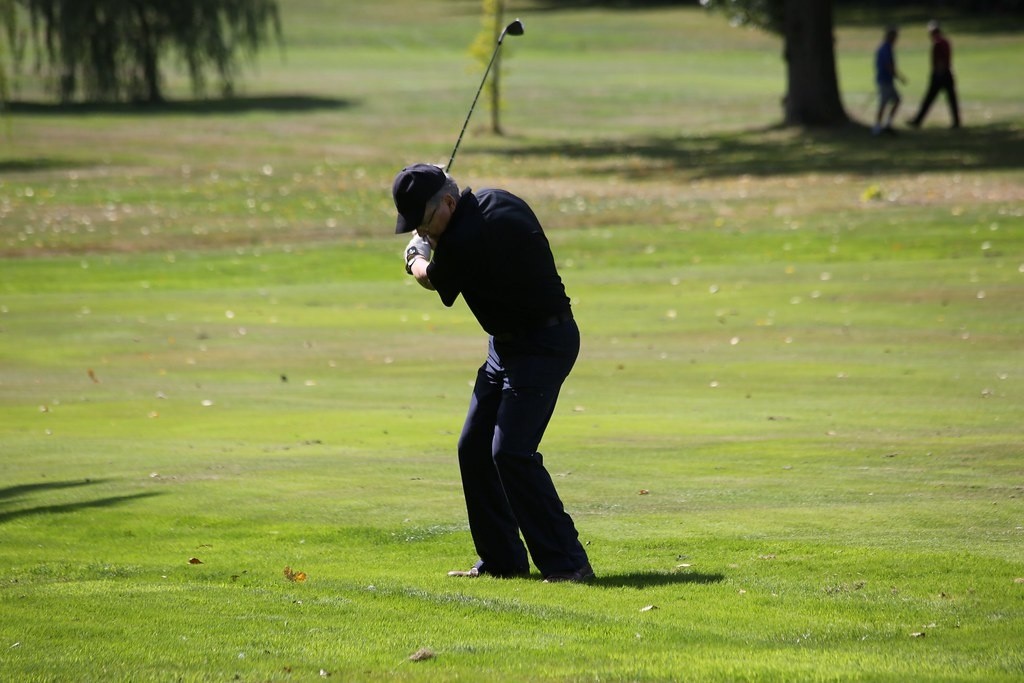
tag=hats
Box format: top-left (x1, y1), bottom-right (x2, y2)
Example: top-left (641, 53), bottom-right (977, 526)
top-left (392, 164), bottom-right (446, 233)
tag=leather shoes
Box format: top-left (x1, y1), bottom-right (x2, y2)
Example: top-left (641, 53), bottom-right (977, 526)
top-left (447, 568), bottom-right (530, 578)
top-left (542, 562), bottom-right (595, 583)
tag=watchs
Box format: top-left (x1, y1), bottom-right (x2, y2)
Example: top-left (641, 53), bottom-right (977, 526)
top-left (408, 255), bottom-right (425, 274)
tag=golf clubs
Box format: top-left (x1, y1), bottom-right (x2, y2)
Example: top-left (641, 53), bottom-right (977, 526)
top-left (445, 17), bottom-right (525, 174)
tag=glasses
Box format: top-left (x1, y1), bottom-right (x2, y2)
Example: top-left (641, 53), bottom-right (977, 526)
top-left (418, 195), bottom-right (444, 232)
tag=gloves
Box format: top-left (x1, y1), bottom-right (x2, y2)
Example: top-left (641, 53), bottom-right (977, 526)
top-left (404, 229), bottom-right (431, 275)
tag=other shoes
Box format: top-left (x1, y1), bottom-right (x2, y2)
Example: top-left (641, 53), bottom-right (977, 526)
top-left (951, 125), bottom-right (959, 129)
top-left (908, 122), bottom-right (918, 129)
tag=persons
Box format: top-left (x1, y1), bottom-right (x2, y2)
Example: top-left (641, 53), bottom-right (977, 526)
top-left (392, 163), bottom-right (597, 584)
top-left (872, 29), bottom-right (907, 137)
top-left (905, 20), bottom-right (961, 131)
